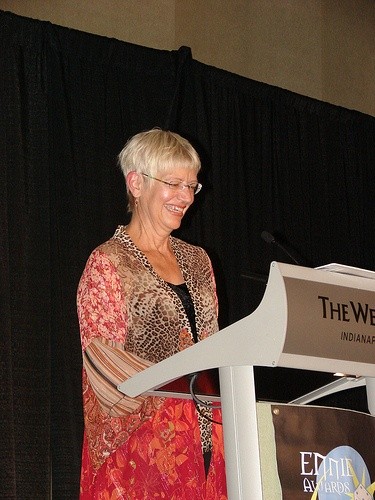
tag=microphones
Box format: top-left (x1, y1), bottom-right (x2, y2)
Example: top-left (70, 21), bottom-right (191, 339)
top-left (261, 230), bottom-right (299, 266)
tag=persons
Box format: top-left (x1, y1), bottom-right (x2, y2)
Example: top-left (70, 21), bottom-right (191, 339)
top-left (76, 127), bottom-right (227, 500)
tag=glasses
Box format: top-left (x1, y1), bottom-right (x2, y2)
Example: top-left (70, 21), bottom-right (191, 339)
top-left (140, 172), bottom-right (202, 195)
